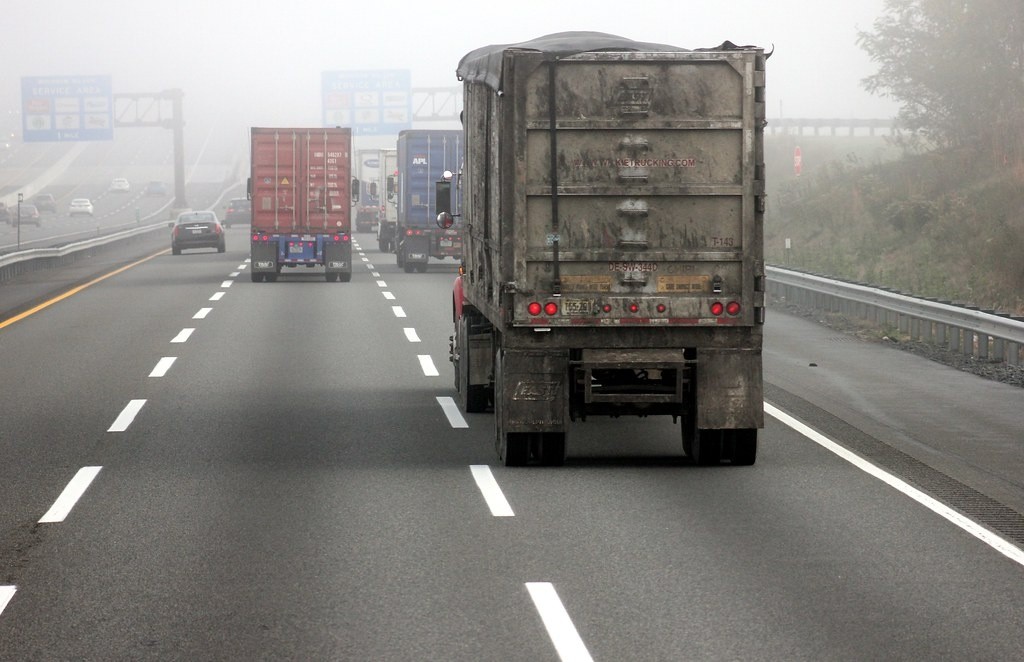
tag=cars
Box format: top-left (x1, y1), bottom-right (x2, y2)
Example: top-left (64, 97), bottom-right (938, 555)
top-left (223, 199), bottom-right (253, 227)
top-left (32, 193), bottom-right (57, 215)
top-left (169, 211), bottom-right (227, 254)
top-left (11, 204), bottom-right (42, 228)
top-left (70, 198), bottom-right (94, 218)
top-left (109, 178), bottom-right (131, 192)
top-left (0, 202), bottom-right (13, 224)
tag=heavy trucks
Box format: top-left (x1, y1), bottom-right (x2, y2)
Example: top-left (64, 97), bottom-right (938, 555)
top-left (433, 31), bottom-right (774, 466)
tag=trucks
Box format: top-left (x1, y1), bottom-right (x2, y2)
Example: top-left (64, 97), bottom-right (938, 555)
top-left (386, 128), bottom-right (465, 272)
top-left (247, 125), bottom-right (360, 282)
top-left (370, 149), bottom-right (399, 255)
top-left (355, 147), bottom-right (382, 233)
